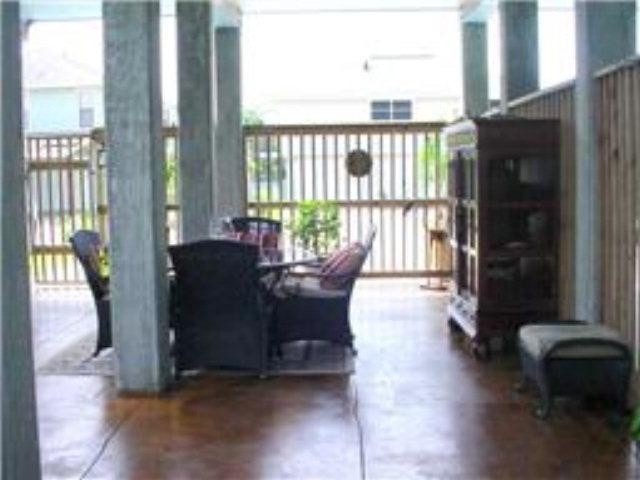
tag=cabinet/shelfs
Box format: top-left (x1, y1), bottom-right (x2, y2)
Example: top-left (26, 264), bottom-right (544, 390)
top-left (441, 117), bottom-right (561, 360)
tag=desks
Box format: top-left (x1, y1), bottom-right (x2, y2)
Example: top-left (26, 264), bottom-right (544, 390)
top-left (256, 244), bottom-right (319, 289)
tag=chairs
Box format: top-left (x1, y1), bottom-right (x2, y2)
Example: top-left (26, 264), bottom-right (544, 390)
top-left (269, 227), bottom-right (377, 364)
top-left (165, 238), bottom-right (271, 377)
top-left (226, 217), bottom-right (283, 245)
top-left (68, 229), bottom-right (178, 360)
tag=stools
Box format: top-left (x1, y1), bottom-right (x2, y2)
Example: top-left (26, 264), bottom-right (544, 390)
top-left (516, 318), bottom-right (632, 421)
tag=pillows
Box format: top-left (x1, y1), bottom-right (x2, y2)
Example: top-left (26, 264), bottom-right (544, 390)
top-left (319, 239), bottom-right (368, 289)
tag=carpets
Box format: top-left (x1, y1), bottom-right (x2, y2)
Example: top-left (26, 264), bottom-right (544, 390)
top-left (34, 331), bottom-right (355, 375)
